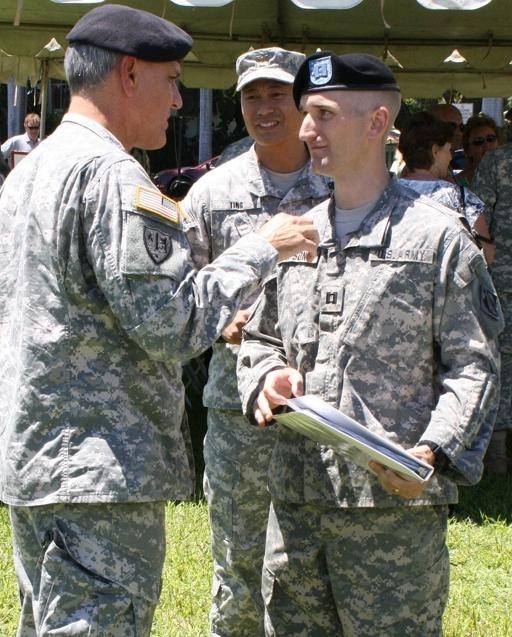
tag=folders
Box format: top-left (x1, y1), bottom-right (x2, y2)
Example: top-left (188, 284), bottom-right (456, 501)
top-left (271, 394), bottom-right (435, 483)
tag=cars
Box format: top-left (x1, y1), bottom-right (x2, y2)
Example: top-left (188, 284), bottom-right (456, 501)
top-left (154, 151), bottom-right (223, 200)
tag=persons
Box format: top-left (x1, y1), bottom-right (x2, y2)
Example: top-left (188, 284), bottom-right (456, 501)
top-left (157, 45), bottom-right (344, 637)
top-left (1, 2), bottom-right (320, 636)
top-left (386, 104), bottom-right (511, 517)
top-left (231, 51), bottom-right (504, 636)
top-left (0, 112), bottom-right (41, 172)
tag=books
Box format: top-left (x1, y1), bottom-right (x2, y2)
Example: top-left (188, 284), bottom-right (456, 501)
top-left (264, 380), bottom-right (436, 483)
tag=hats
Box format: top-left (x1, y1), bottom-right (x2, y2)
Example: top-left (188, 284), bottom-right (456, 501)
top-left (292, 48), bottom-right (404, 110)
top-left (231, 47), bottom-right (308, 92)
top-left (65, 3), bottom-right (194, 64)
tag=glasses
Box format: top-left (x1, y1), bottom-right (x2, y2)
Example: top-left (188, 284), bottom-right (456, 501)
top-left (445, 120), bottom-right (465, 132)
top-left (467, 134), bottom-right (497, 146)
top-left (27, 125), bottom-right (40, 130)
